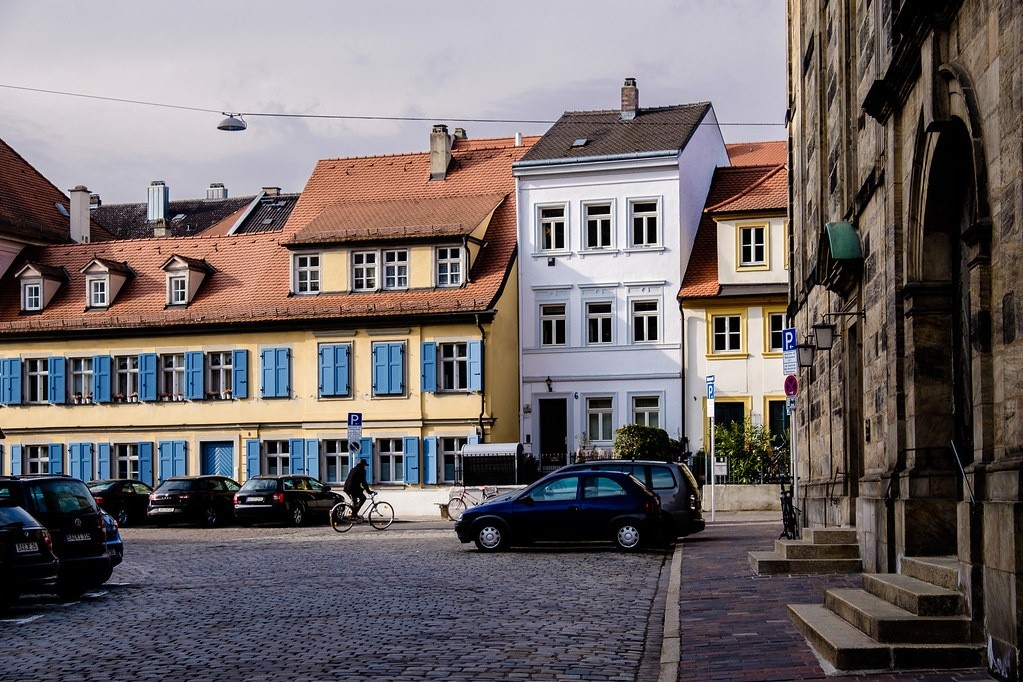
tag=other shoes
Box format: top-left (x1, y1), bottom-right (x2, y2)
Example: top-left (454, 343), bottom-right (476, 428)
top-left (351, 514), bottom-right (361, 520)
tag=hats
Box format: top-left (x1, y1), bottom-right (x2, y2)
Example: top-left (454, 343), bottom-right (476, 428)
top-left (360, 459), bottom-right (369, 467)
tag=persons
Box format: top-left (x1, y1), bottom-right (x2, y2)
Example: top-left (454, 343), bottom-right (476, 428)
top-left (342, 458), bottom-right (375, 517)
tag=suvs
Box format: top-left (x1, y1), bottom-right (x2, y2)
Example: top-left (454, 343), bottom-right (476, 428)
top-left (475, 456), bottom-right (706, 543)
top-left (454, 470), bottom-right (661, 554)
top-left (0, 473), bottom-right (124, 598)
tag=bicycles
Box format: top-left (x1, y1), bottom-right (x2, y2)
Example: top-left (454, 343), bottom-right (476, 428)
top-left (328, 490), bottom-right (395, 533)
top-left (445, 481), bottom-right (499, 523)
top-left (776, 478), bottom-right (797, 541)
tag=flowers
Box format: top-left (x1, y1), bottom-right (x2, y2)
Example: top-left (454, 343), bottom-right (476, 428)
top-left (71, 394), bottom-right (81, 400)
top-left (160, 392), bottom-right (183, 397)
top-left (85, 392), bottom-right (93, 399)
top-left (224, 389), bottom-right (232, 394)
top-left (114, 393), bottom-right (124, 399)
top-left (128, 392), bottom-right (138, 398)
top-left (206, 390), bottom-right (219, 396)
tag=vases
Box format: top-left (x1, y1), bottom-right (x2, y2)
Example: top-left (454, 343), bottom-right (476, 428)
top-left (86, 399), bottom-right (90, 404)
top-left (173, 396), bottom-right (177, 401)
top-left (118, 399), bottom-right (122, 403)
top-left (75, 399), bottom-right (78, 404)
top-left (178, 396), bottom-right (183, 401)
top-left (227, 394), bottom-right (230, 399)
top-left (162, 396), bottom-right (168, 401)
top-left (133, 397), bottom-right (137, 402)
top-left (212, 396), bottom-right (216, 400)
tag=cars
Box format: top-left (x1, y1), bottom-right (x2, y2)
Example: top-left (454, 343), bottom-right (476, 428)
top-left (231, 472), bottom-right (346, 528)
top-left (83, 478), bottom-right (155, 528)
top-left (0, 499), bottom-right (61, 606)
top-left (146, 474), bottom-right (245, 528)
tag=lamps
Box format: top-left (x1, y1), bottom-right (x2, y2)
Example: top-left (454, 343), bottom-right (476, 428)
top-left (810, 304), bottom-right (866, 350)
top-left (216, 112), bottom-right (246, 131)
top-left (546, 376), bottom-right (552, 392)
top-left (795, 334), bottom-right (840, 367)
